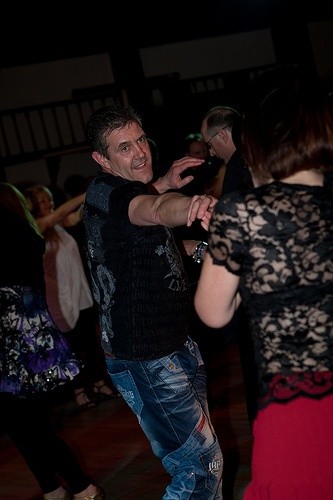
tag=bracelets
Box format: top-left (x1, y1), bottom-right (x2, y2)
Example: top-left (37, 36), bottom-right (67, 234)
top-left (193, 241), bottom-right (208, 263)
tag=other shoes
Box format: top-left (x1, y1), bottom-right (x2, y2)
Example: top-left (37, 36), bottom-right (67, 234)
top-left (44, 489), bottom-right (70, 500)
top-left (72, 482), bottom-right (104, 500)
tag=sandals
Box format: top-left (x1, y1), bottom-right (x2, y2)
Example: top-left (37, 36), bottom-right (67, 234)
top-left (73, 391), bottom-right (97, 408)
top-left (92, 384), bottom-right (121, 397)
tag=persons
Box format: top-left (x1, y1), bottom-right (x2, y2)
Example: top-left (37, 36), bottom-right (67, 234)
top-left (81, 104), bottom-right (223, 500)
top-left (194, 82), bottom-right (333, 500)
top-left (26, 106), bottom-right (254, 410)
top-left (0, 183), bottom-right (105, 500)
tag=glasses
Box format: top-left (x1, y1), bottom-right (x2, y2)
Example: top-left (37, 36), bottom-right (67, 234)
top-left (206, 126), bottom-right (228, 150)
